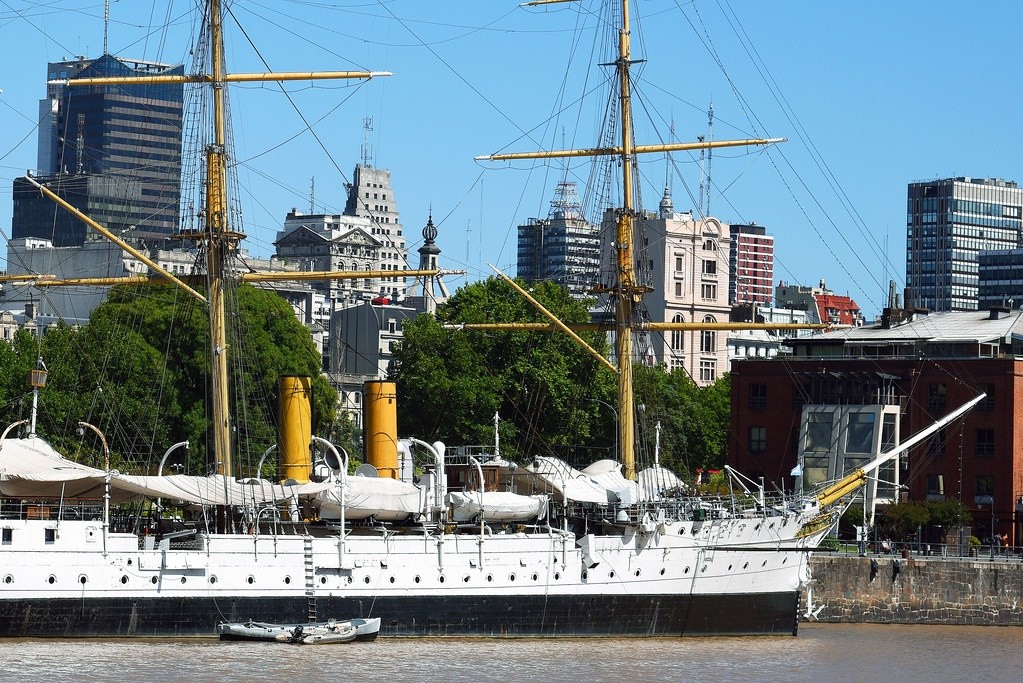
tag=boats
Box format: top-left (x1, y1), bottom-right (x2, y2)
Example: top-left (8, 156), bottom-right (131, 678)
top-left (218, 616), bottom-right (380, 645)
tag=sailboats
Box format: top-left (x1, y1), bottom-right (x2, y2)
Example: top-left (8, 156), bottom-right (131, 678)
top-left (0, 0), bottom-right (986, 639)
